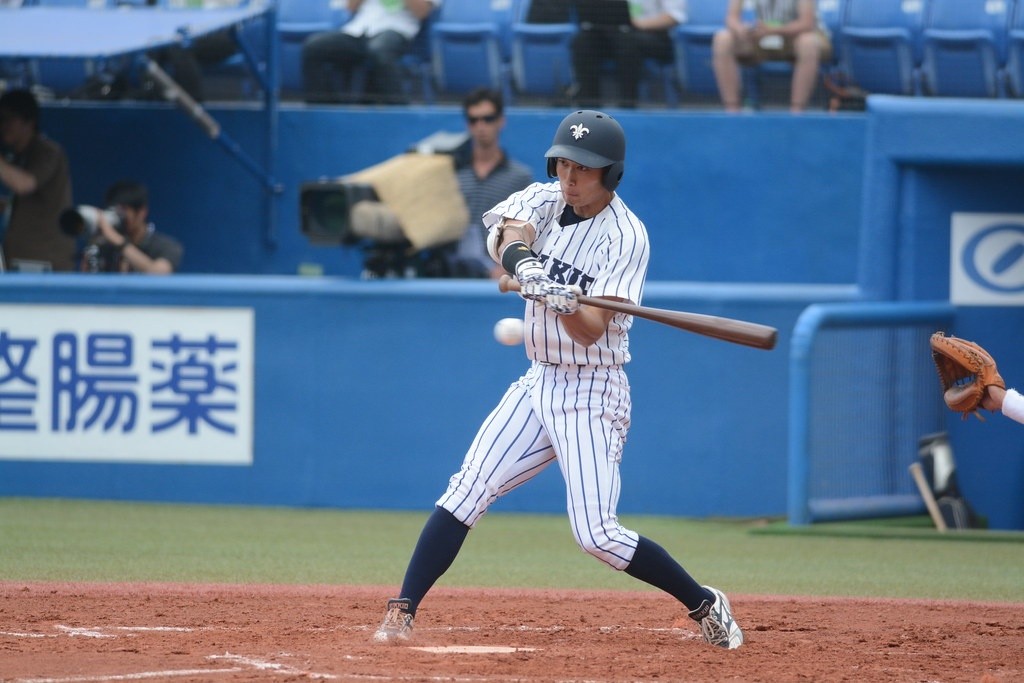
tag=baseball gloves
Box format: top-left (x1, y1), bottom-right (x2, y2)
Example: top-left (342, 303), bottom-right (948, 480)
top-left (928, 331), bottom-right (1006, 413)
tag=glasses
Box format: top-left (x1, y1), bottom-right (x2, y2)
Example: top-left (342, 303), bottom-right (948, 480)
top-left (467, 114), bottom-right (497, 122)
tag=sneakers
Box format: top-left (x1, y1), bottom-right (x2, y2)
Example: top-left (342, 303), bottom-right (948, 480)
top-left (373, 598), bottom-right (415, 641)
top-left (688, 585), bottom-right (743, 649)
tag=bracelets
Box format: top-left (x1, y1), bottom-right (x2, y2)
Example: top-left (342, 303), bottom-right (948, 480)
top-left (117, 234), bottom-right (131, 250)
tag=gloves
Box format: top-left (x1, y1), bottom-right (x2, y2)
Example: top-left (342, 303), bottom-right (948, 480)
top-left (545, 280), bottom-right (582, 315)
top-left (515, 256), bottom-right (552, 302)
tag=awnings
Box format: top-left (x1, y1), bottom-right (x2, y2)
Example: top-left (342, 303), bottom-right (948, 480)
top-left (0, 6), bottom-right (270, 61)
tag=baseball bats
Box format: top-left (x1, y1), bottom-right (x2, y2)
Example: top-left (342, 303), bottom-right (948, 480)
top-left (498, 274), bottom-right (778, 350)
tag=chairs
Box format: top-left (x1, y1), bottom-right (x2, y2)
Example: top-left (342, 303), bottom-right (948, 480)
top-left (1, 1), bottom-right (1024, 111)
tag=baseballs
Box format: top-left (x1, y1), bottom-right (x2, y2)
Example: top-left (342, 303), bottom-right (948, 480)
top-left (493, 318), bottom-right (524, 346)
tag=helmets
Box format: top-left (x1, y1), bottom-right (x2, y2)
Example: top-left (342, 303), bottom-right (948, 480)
top-left (544, 110), bottom-right (626, 192)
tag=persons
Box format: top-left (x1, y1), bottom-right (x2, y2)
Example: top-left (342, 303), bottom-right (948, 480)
top-left (710, 0), bottom-right (844, 113)
top-left (0, 87), bottom-right (78, 271)
top-left (930, 332), bottom-right (1024, 426)
top-left (428, 86), bottom-right (536, 279)
top-left (374, 111), bottom-right (744, 652)
top-left (302, 0), bottom-right (443, 104)
top-left (79, 182), bottom-right (182, 275)
top-left (568, 0), bottom-right (688, 109)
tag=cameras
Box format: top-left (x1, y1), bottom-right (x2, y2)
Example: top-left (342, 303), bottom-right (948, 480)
top-left (60, 205), bottom-right (123, 236)
